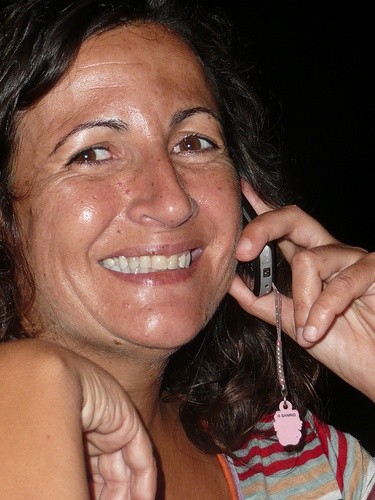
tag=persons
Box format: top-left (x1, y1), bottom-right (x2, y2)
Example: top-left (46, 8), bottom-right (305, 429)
top-left (0, 0), bottom-right (374, 499)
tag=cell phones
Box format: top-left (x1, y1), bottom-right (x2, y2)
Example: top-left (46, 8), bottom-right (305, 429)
top-left (236, 191), bottom-right (274, 296)
